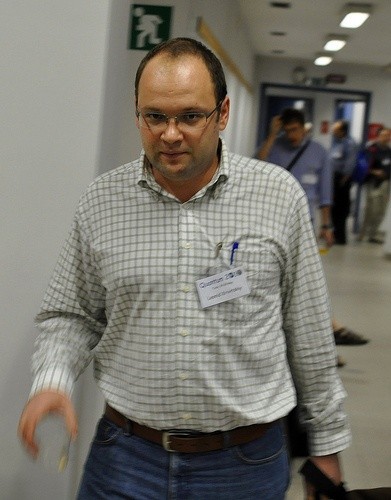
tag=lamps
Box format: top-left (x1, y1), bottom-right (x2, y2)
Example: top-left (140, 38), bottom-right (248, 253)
top-left (336, 3), bottom-right (373, 29)
top-left (314, 52), bottom-right (335, 66)
top-left (321, 34), bottom-right (350, 51)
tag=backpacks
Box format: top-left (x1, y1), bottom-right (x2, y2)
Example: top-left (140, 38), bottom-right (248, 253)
top-left (352, 152), bottom-right (368, 181)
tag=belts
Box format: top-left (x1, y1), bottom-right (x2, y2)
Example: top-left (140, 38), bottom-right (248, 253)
top-left (104, 403), bottom-right (286, 452)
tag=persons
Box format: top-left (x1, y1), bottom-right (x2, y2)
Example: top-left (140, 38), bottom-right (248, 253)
top-left (327, 120), bottom-right (391, 246)
top-left (17, 38), bottom-right (350, 500)
top-left (248, 108), bottom-right (333, 245)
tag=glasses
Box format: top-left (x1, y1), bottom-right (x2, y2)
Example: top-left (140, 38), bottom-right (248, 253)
top-left (137, 98), bottom-right (224, 130)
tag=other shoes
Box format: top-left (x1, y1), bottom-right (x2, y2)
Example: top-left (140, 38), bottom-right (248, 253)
top-left (338, 357), bottom-right (344, 366)
top-left (334, 327), bottom-right (367, 344)
top-left (369, 237), bottom-right (383, 244)
